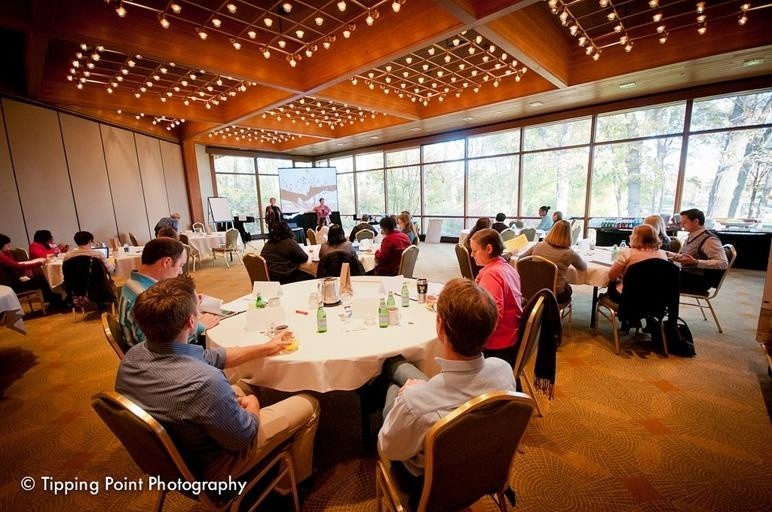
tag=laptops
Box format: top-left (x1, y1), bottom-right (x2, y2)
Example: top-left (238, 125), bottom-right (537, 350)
top-left (92, 247), bottom-right (109, 258)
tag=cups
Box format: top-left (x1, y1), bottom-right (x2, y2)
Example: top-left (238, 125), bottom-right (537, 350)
top-left (268, 296), bottom-right (280, 307)
top-left (308, 293), bottom-right (319, 310)
top-left (268, 324), bottom-right (289, 339)
top-left (388, 306), bottom-right (402, 326)
top-left (417, 277), bottom-right (428, 303)
top-left (280, 331), bottom-right (298, 354)
top-left (575, 239), bottom-right (595, 259)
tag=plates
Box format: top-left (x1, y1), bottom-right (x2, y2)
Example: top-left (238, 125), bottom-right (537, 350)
top-left (425, 293), bottom-right (443, 314)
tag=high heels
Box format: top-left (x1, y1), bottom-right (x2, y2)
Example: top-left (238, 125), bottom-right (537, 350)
top-left (621, 319), bottom-right (630, 335)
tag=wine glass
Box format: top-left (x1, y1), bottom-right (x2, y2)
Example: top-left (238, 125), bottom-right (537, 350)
top-left (336, 295), bottom-right (354, 333)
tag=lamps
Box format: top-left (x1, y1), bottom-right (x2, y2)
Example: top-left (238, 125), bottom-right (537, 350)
top-left (66, 1), bottom-right (751, 145)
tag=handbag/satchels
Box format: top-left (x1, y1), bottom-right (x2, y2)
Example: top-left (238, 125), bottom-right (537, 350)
top-left (64, 294), bottom-right (90, 309)
top-left (664, 320), bottom-right (696, 357)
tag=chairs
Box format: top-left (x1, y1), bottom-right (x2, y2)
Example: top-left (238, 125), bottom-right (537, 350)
top-left (1, 220), bottom-right (737, 512)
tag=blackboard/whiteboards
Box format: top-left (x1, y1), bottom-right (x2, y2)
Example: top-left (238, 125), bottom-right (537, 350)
top-left (208, 196), bottom-right (234, 223)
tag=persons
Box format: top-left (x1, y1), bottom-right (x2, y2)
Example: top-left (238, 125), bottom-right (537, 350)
top-left (0, 197), bottom-right (729, 369)
top-left (115, 271), bottom-right (322, 512)
top-left (369, 277), bottom-right (517, 512)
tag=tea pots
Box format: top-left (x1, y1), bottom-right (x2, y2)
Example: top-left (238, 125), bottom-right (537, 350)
top-left (317, 277), bottom-right (341, 306)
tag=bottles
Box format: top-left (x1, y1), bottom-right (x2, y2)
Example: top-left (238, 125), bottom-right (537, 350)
top-left (600, 217), bottom-right (645, 230)
top-left (123, 243), bottom-right (129, 255)
top-left (388, 291), bottom-right (396, 307)
top-left (254, 291), bottom-right (266, 308)
top-left (378, 297), bottom-right (389, 327)
top-left (352, 238), bottom-right (361, 256)
top-left (317, 296), bottom-right (328, 334)
top-left (401, 281), bottom-right (411, 308)
top-left (610, 239), bottom-right (626, 264)
